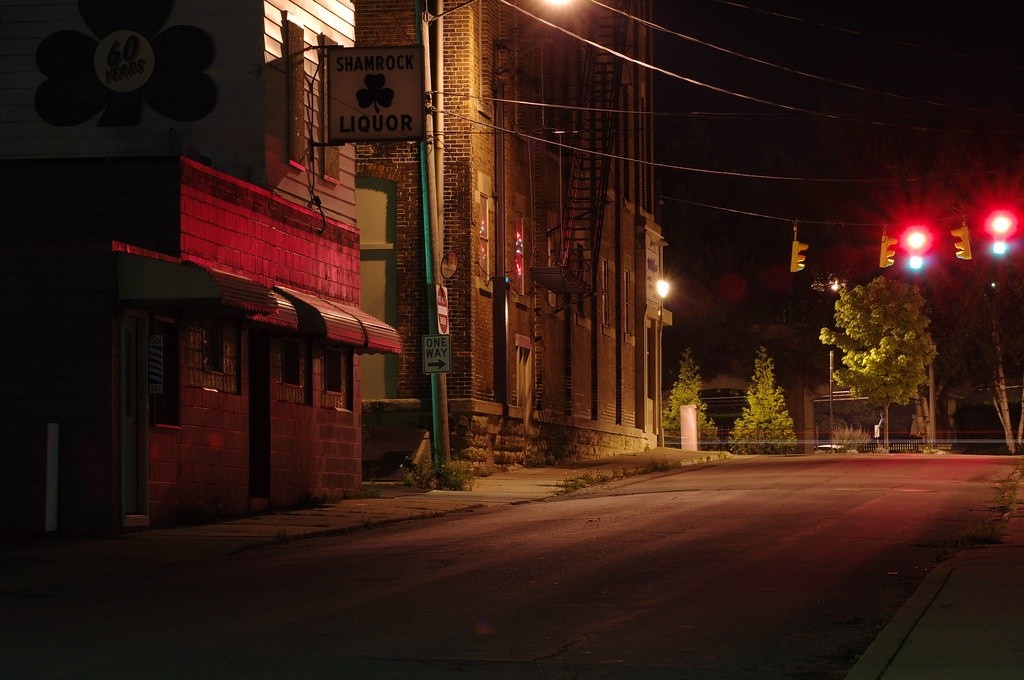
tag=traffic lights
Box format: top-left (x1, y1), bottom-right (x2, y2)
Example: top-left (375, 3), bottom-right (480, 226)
top-left (992, 218), bottom-right (1007, 254)
top-left (791, 241), bottom-right (809, 272)
top-left (909, 233), bottom-right (924, 270)
top-left (951, 227), bottom-right (972, 260)
top-left (879, 236), bottom-right (899, 268)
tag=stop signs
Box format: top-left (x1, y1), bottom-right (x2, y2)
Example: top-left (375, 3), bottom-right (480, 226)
top-left (436, 283), bottom-right (450, 335)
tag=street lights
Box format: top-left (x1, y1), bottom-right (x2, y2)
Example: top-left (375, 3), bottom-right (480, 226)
top-left (657, 282), bottom-right (669, 446)
top-left (417, 0), bottom-right (577, 493)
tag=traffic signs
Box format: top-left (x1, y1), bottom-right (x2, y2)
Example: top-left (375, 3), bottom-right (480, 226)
top-left (423, 334), bottom-right (452, 375)
